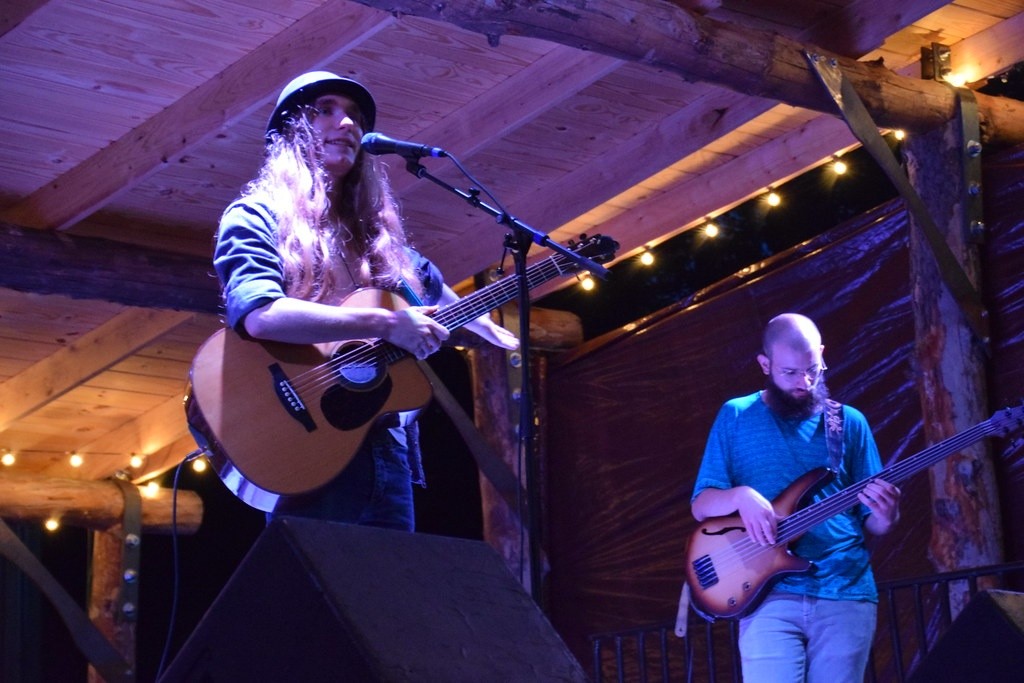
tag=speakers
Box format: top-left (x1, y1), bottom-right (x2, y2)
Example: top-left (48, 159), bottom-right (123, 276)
top-left (158, 515), bottom-right (588, 683)
top-left (905, 588), bottom-right (1024, 683)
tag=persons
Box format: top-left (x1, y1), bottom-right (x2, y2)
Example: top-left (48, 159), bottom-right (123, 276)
top-left (213, 72), bottom-right (521, 533)
top-left (689, 311), bottom-right (903, 682)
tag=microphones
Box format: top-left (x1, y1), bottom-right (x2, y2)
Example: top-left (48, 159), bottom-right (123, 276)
top-left (361, 132), bottom-right (447, 158)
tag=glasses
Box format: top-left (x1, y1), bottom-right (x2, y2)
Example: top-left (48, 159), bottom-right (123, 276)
top-left (769, 351), bottom-right (827, 381)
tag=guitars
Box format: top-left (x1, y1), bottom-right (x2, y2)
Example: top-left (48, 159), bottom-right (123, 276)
top-left (184, 232), bottom-right (624, 512)
top-left (684, 403), bottom-right (1024, 622)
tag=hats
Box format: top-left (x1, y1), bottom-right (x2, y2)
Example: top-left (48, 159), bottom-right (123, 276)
top-left (264, 69), bottom-right (376, 152)
